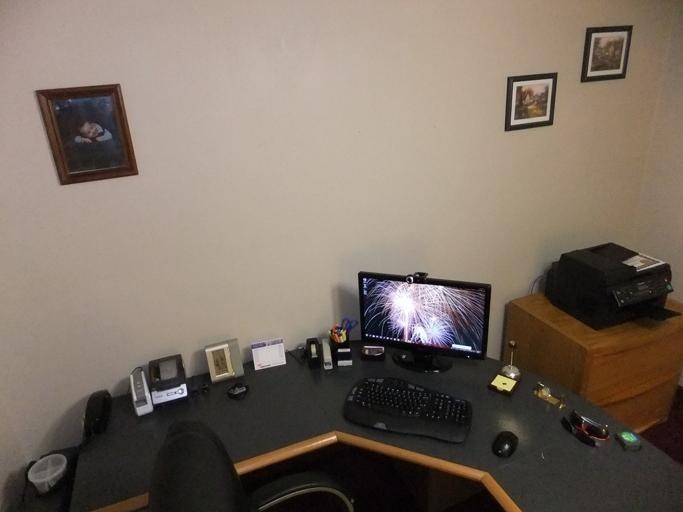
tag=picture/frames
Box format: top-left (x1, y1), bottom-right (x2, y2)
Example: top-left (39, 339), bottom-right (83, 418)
top-left (34, 84), bottom-right (138, 185)
top-left (505, 73), bottom-right (557, 131)
top-left (579, 25), bottom-right (632, 82)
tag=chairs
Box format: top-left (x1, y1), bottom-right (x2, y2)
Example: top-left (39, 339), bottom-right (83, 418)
top-left (148, 421), bottom-right (364, 512)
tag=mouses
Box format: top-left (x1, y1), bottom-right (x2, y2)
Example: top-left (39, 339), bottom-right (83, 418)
top-left (492, 430), bottom-right (518, 458)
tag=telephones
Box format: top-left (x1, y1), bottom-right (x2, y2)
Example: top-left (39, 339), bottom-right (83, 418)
top-left (83, 391), bottom-right (111, 436)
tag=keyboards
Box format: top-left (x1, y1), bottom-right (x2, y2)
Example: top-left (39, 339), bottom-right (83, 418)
top-left (343, 377), bottom-right (473, 444)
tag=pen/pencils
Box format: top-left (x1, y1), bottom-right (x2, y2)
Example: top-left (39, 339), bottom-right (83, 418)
top-left (329, 325), bottom-right (346, 342)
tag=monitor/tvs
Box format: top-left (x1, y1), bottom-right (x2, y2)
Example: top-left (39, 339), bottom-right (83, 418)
top-left (356, 270), bottom-right (491, 374)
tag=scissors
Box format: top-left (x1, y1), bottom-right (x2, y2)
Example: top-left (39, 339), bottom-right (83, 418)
top-left (341, 317), bottom-right (358, 335)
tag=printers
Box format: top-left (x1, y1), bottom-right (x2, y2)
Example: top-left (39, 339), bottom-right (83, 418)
top-left (545, 241), bottom-right (681, 331)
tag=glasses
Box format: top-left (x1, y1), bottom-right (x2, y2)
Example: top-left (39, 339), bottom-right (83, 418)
top-left (561, 416), bottom-right (597, 446)
top-left (571, 409), bottom-right (606, 437)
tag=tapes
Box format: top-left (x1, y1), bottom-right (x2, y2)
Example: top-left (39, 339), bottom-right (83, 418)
top-left (311, 344), bottom-right (318, 358)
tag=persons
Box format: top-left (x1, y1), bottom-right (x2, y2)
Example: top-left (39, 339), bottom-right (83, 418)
top-left (71, 113), bottom-right (119, 145)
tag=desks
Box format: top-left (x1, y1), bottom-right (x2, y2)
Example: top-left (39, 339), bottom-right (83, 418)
top-left (63, 341), bottom-right (682, 511)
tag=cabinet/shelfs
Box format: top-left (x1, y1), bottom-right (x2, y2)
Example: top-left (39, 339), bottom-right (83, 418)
top-left (500, 302), bottom-right (683, 436)
